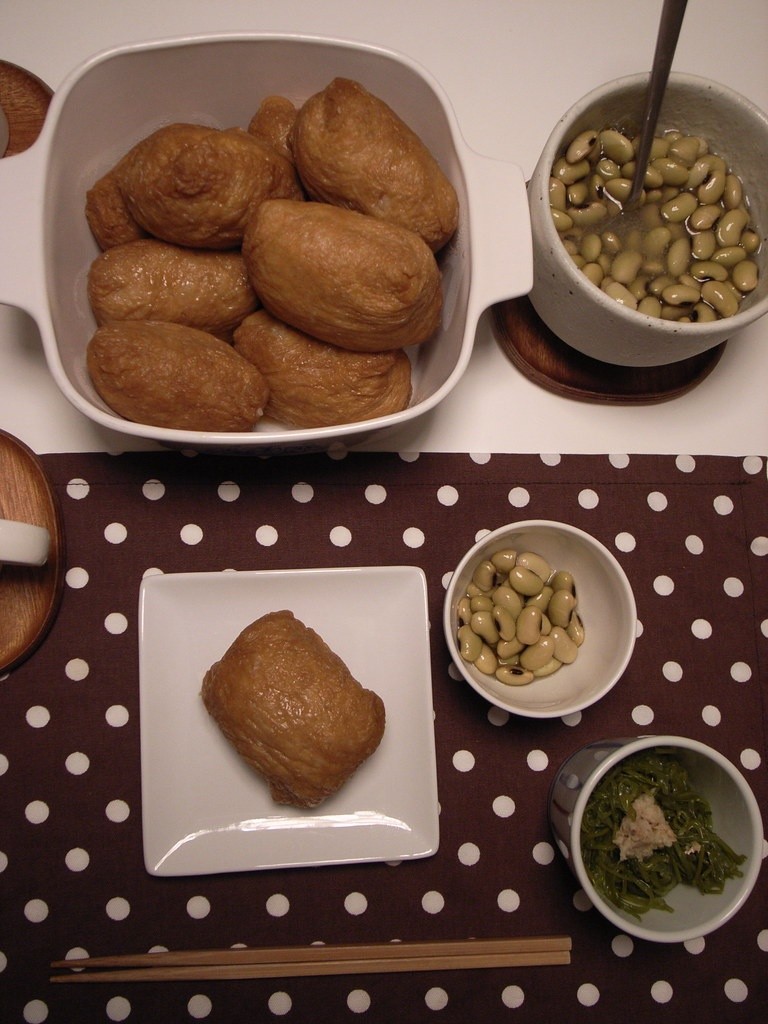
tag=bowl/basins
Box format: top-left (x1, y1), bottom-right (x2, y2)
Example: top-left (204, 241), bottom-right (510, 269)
top-left (545, 735), bottom-right (764, 942)
top-left (0, 31), bottom-right (534, 442)
top-left (444, 520), bottom-right (637, 718)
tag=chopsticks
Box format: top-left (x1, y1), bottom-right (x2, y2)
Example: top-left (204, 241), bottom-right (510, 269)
top-left (50, 918), bottom-right (573, 984)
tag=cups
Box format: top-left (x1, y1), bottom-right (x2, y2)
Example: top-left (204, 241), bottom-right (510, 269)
top-left (527, 70), bottom-right (768, 368)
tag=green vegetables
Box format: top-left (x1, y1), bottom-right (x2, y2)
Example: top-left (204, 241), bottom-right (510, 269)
top-left (578, 748), bottom-right (746, 918)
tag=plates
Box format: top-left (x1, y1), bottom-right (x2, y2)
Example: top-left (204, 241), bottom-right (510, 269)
top-left (138, 565), bottom-right (440, 875)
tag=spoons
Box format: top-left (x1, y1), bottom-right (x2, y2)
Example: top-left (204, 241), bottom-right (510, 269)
top-left (597, 0), bottom-right (688, 234)
